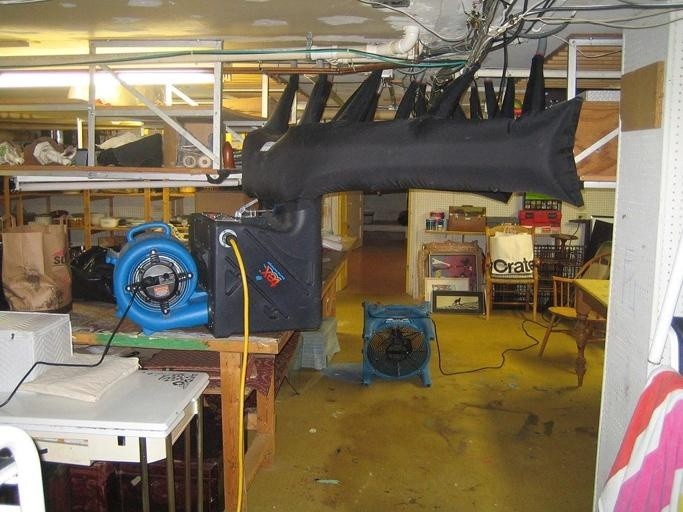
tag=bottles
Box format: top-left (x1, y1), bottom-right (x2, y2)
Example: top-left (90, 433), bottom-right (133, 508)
top-left (437, 219), bottom-right (447, 231)
top-left (223, 140), bottom-right (235, 170)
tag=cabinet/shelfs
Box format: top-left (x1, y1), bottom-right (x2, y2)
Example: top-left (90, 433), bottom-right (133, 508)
top-left (0, 38), bottom-right (267, 251)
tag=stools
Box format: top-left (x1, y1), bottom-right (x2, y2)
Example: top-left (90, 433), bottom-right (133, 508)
top-left (298, 315), bottom-right (341, 371)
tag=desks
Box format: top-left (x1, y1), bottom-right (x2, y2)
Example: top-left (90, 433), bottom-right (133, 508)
top-left (0, 370), bottom-right (210, 511)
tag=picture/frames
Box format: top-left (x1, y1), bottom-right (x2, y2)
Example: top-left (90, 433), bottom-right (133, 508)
top-left (423, 245), bottom-right (485, 316)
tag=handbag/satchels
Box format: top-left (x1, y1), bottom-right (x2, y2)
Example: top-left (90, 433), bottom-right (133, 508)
top-left (1, 214), bottom-right (74, 312)
top-left (489, 224), bottom-right (534, 279)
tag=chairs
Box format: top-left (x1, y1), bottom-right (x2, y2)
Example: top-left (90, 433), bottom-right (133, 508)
top-left (485, 222), bottom-right (612, 361)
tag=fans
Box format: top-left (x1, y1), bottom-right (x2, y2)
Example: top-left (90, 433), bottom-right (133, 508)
top-left (359, 300), bottom-right (436, 386)
